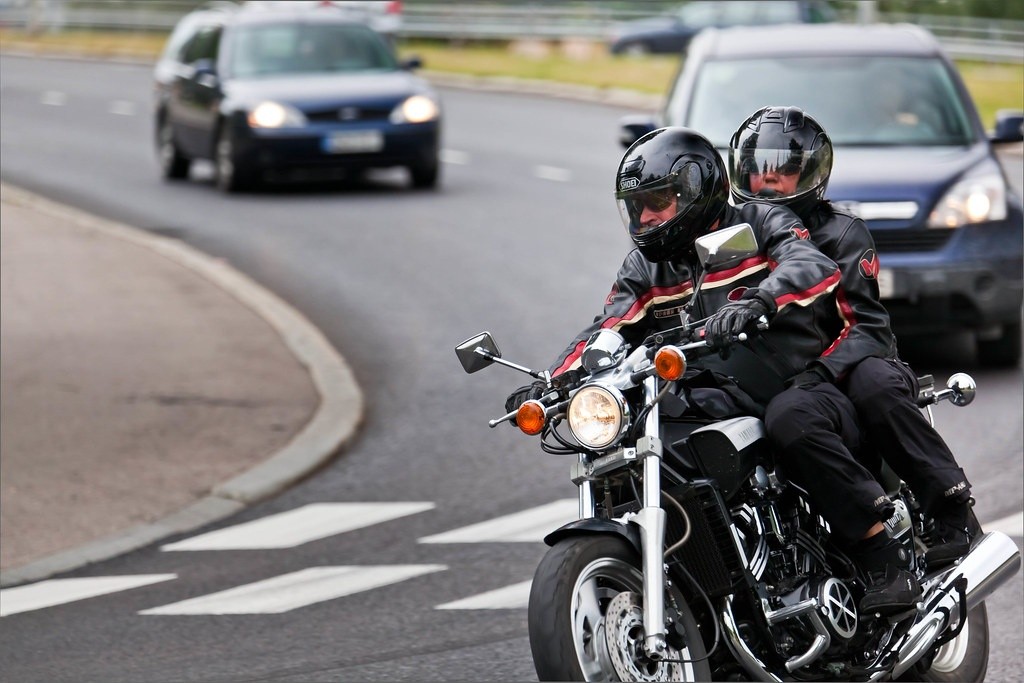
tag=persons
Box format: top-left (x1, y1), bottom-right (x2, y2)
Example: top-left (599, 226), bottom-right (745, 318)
top-left (504, 105), bottom-right (984, 615)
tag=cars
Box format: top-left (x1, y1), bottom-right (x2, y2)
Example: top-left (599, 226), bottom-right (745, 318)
top-left (610, 0), bottom-right (843, 60)
top-left (616, 20), bottom-right (1024, 376)
top-left (150, 5), bottom-right (444, 193)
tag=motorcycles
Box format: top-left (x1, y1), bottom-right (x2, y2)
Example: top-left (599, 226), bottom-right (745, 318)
top-left (452, 218), bottom-right (1023, 682)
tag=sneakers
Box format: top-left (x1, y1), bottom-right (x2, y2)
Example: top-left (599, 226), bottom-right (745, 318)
top-left (859, 538), bottom-right (924, 616)
top-left (924, 496), bottom-right (984, 570)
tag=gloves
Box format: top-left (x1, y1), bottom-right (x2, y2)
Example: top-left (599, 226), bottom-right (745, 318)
top-left (705, 289), bottom-right (779, 360)
top-left (783, 365), bottom-right (832, 392)
top-left (505, 382), bottom-right (546, 428)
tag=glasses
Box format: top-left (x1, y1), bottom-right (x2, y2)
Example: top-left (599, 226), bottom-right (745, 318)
top-left (626, 188), bottom-right (677, 218)
top-left (744, 158), bottom-right (808, 176)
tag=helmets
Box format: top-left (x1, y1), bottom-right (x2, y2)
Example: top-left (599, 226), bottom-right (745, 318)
top-left (614, 127), bottom-right (729, 263)
top-left (729, 105), bottom-right (833, 219)
